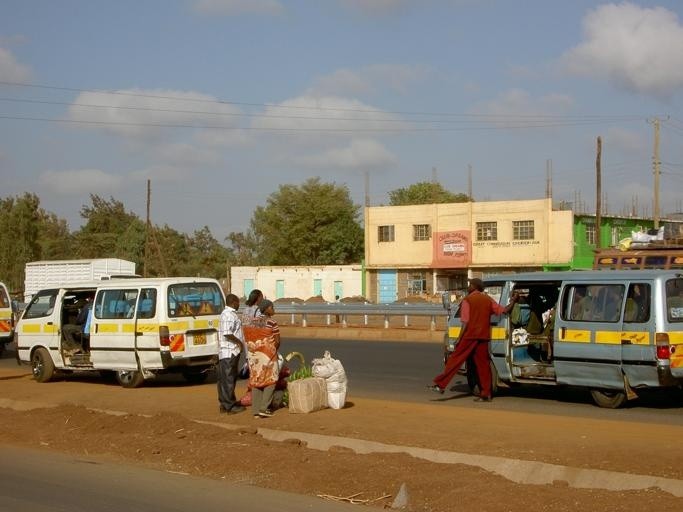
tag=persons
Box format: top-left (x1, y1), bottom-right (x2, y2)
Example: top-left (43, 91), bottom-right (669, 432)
top-left (328, 293), bottom-right (341, 304)
top-left (606, 287), bottom-right (638, 323)
top-left (59, 293), bottom-right (101, 356)
top-left (423, 277), bottom-right (520, 402)
top-left (217, 293), bottom-right (248, 414)
top-left (561, 286), bottom-right (595, 320)
top-left (246, 300), bottom-right (280, 418)
top-left (242, 289), bottom-right (284, 379)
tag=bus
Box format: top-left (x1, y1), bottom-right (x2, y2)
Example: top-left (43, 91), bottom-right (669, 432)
top-left (592, 237), bottom-right (683, 320)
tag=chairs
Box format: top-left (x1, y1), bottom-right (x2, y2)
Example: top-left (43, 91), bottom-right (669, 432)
top-left (106, 299), bottom-right (129, 313)
top-left (138, 299), bottom-right (154, 312)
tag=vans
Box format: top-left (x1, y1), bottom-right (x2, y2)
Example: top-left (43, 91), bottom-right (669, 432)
top-left (0, 281), bottom-right (14, 350)
top-left (443, 272), bottom-right (683, 409)
top-left (14, 277), bottom-right (228, 388)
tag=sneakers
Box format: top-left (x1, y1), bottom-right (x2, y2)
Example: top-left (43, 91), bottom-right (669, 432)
top-left (253, 408), bottom-right (275, 419)
top-left (64, 349), bottom-right (80, 357)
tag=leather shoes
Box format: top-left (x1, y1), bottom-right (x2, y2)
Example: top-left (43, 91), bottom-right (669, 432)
top-left (220, 401), bottom-right (244, 414)
top-left (474, 397), bottom-right (490, 402)
top-left (425, 384), bottom-right (444, 394)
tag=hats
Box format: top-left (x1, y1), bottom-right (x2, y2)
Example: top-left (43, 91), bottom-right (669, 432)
top-left (466, 278), bottom-right (485, 292)
